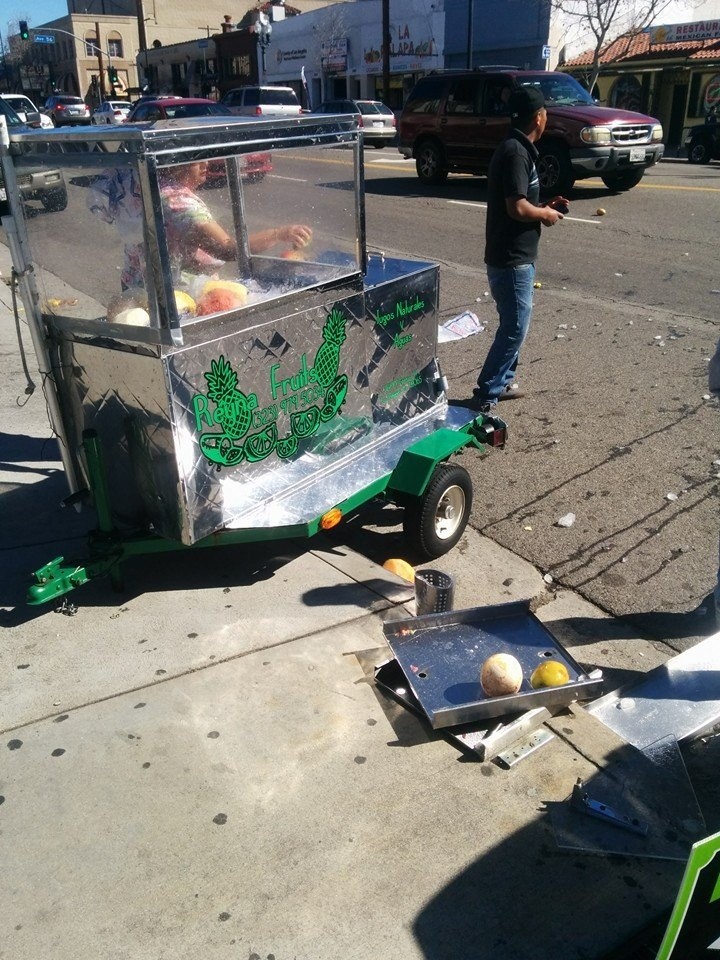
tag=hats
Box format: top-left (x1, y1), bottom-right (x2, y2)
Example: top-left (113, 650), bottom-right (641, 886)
top-left (509, 85), bottom-right (552, 117)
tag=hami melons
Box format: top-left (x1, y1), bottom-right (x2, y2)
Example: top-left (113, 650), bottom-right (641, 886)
top-left (173, 290), bottom-right (197, 315)
top-left (382, 558), bottom-right (416, 583)
top-left (480, 654), bottom-right (523, 697)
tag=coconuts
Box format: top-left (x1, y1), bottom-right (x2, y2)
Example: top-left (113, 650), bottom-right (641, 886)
top-left (105, 288), bottom-right (151, 327)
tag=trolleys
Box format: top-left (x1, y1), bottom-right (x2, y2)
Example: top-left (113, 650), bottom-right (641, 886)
top-left (1, 111), bottom-right (509, 560)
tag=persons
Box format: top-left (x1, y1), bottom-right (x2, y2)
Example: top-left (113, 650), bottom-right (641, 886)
top-left (87, 161), bottom-right (312, 292)
top-left (460, 88), bottom-right (569, 410)
top-left (707, 106), bottom-right (717, 124)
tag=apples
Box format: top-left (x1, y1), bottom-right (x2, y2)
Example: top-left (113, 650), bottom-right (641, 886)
top-left (531, 661), bottom-right (569, 690)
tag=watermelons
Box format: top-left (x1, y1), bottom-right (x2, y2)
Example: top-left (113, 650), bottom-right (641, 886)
top-left (198, 288), bottom-right (243, 318)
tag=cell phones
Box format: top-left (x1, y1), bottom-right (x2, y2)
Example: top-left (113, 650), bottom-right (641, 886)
top-left (552, 203), bottom-right (569, 215)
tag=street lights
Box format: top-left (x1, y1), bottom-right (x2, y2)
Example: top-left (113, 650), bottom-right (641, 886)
top-left (255, 17), bottom-right (273, 85)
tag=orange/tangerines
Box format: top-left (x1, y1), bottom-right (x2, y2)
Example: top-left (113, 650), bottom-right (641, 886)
top-left (596, 208), bottom-right (606, 215)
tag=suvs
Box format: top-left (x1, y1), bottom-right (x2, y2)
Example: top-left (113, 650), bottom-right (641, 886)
top-left (312, 98), bottom-right (396, 149)
top-left (396, 66), bottom-right (666, 196)
top-left (1, 94), bottom-right (68, 211)
top-left (39, 95), bottom-right (92, 128)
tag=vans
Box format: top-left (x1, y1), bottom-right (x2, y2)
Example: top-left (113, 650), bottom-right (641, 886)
top-left (222, 86), bottom-right (302, 117)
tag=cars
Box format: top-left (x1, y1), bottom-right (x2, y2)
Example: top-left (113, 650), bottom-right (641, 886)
top-left (89, 101), bottom-right (135, 124)
top-left (123, 96), bottom-right (273, 184)
top-left (684, 101), bottom-right (720, 164)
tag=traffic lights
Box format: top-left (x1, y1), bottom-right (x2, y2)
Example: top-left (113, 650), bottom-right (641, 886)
top-left (19, 21), bottom-right (30, 40)
top-left (111, 69), bottom-right (118, 82)
top-left (51, 80), bottom-right (56, 87)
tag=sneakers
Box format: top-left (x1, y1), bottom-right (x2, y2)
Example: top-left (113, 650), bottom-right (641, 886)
top-left (480, 401), bottom-right (496, 416)
top-left (500, 386), bottom-right (525, 400)
